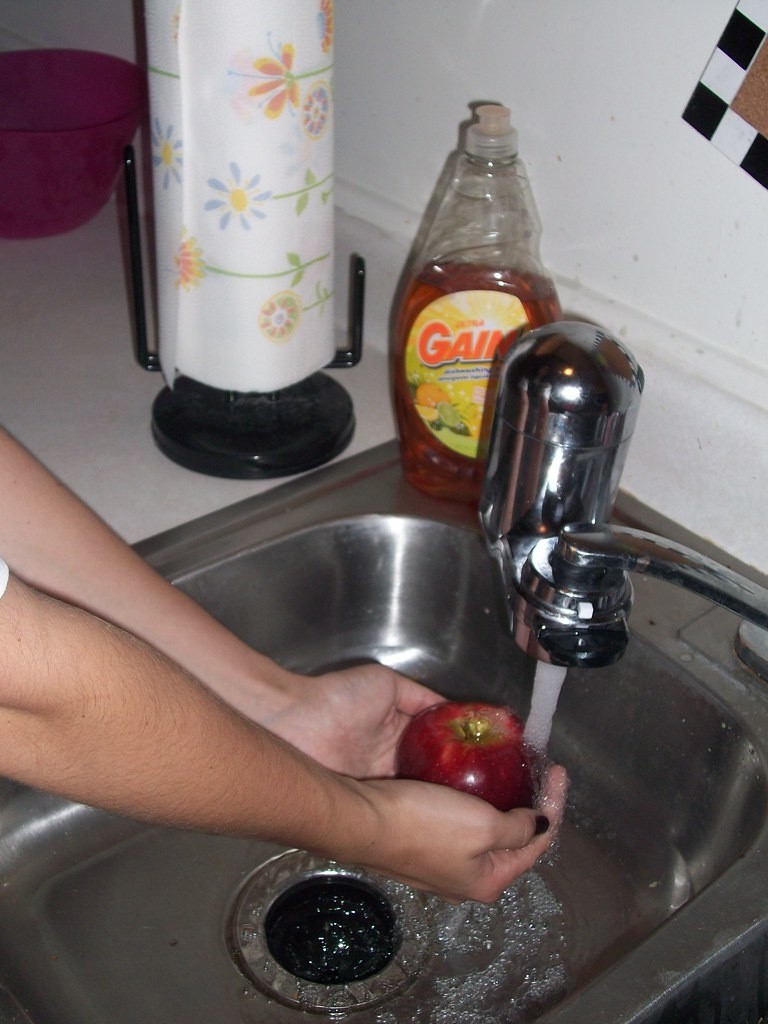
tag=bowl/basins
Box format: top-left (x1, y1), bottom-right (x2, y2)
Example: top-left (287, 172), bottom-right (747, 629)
top-left (1, 46), bottom-right (148, 242)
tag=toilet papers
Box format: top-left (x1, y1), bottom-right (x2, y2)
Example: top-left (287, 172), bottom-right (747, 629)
top-left (141, 0), bottom-right (339, 394)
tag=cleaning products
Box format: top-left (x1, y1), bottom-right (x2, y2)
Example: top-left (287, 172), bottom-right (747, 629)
top-left (387, 105), bottom-right (566, 504)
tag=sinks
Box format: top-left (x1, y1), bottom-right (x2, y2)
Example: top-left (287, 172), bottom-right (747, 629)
top-left (0, 431), bottom-right (768, 1024)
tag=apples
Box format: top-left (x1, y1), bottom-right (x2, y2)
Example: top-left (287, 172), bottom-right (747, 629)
top-left (394, 701), bottom-right (541, 813)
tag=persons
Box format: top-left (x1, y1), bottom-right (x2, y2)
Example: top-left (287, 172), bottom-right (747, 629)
top-left (4, 423), bottom-right (579, 916)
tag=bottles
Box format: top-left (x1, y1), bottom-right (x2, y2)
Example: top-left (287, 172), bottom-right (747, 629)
top-left (388, 101), bottom-right (560, 505)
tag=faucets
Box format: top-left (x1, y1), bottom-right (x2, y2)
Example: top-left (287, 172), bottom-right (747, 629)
top-left (553, 520), bottom-right (768, 635)
